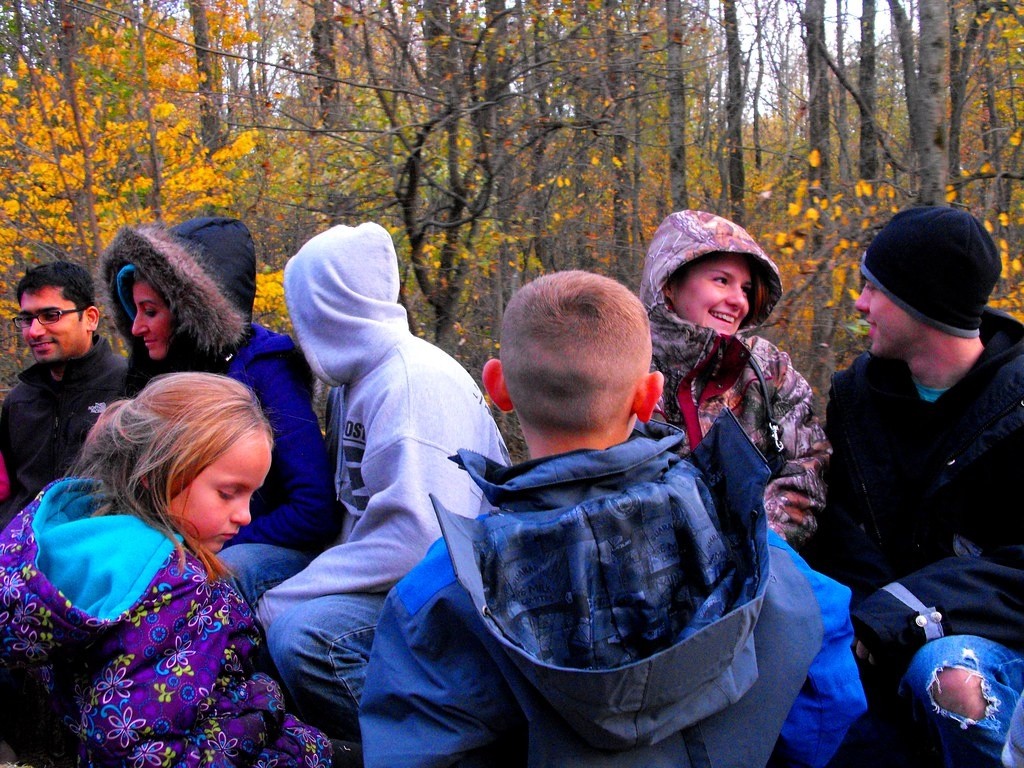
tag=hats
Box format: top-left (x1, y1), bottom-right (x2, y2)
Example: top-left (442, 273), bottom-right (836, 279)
top-left (860, 201), bottom-right (1002, 338)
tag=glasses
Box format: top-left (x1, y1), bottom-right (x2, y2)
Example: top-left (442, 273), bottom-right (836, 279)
top-left (13, 307), bottom-right (85, 329)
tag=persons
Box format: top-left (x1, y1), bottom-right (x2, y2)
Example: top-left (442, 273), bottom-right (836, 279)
top-left (97, 217), bottom-right (335, 553)
top-left (0, 373), bottom-right (335, 767)
top-left (634, 207), bottom-right (833, 550)
top-left (208, 220), bottom-right (513, 739)
top-left (799, 207), bottom-right (1024, 768)
top-left (0, 260), bottom-right (132, 535)
top-left (358, 269), bottom-right (868, 768)
